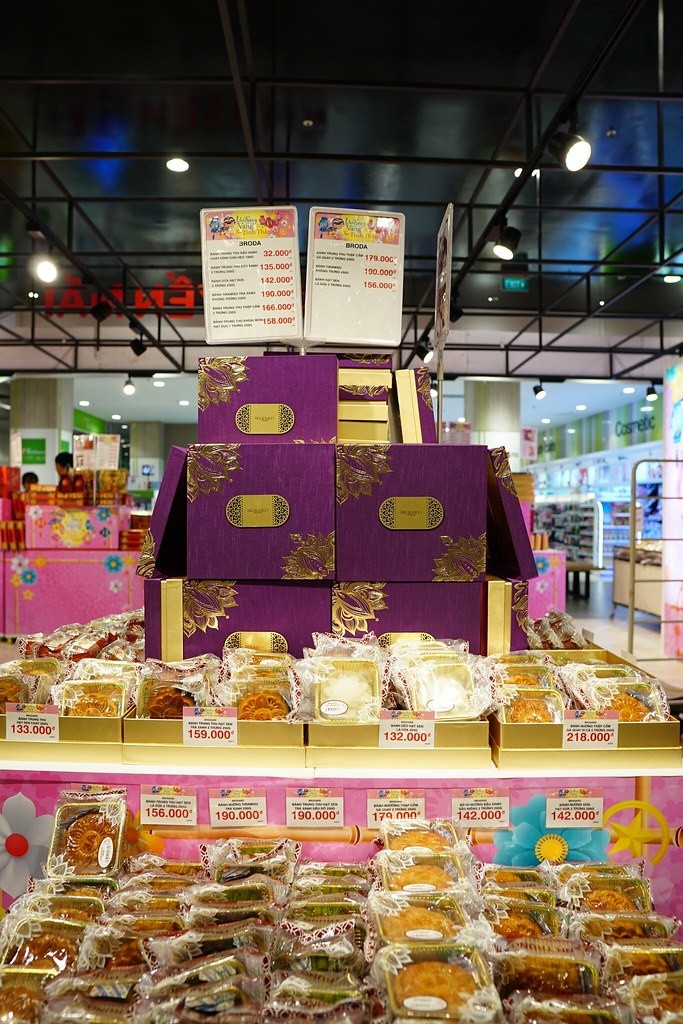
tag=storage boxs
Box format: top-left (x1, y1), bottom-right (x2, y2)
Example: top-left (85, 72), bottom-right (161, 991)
top-left (487, 445), bottom-right (539, 580)
top-left (136, 446), bottom-right (187, 579)
top-left (331, 576), bottom-right (512, 658)
top-left (124, 706), bottom-right (304, 768)
top-left (144, 578), bottom-right (161, 662)
top-left (161, 579), bottom-right (331, 664)
top-left (304, 715), bottom-right (491, 768)
top-left (0, 702), bottom-right (136, 762)
top-left (339, 385), bottom-right (389, 421)
top-left (395, 367), bottom-right (437, 444)
top-left (335, 444), bottom-right (488, 582)
top-left (338, 421), bottom-right (394, 442)
top-left (512, 580), bottom-right (531, 653)
top-left (198, 355), bottom-right (339, 445)
top-left (187, 444), bottom-right (336, 582)
top-left (263, 350), bottom-right (394, 388)
top-left (486, 649), bottom-right (682, 768)
top-left (0, 466), bottom-right (153, 550)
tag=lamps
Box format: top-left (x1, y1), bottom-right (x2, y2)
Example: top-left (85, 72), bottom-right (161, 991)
top-left (88, 297), bottom-right (114, 321)
top-left (128, 335), bottom-right (147, 355)
top-left (548, 114), bottom-right (591, 172)
top-left (532, 381), bottom-right (546, 400)
top-left (416, 338), bottom-right (436, 365)
top-left (122, 376), bottom-right (137, 396)
top-left (36, 244), bottom-right (57, 283)
top-left (646, 384), bottom-right (659, 402)
top-left (492, 223), bottom-right (521, 261)
top-left (450, 294), bottom-right (465, 323)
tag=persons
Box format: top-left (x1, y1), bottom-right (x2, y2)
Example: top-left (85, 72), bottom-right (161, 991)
top-left (22, 451), bottom-right (74, 492)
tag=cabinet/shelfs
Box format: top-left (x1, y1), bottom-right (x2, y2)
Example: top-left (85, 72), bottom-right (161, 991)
top-left (536, 492), bottom-right (639, 568)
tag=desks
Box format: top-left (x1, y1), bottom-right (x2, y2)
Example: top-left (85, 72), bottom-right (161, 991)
top-left (4, 551), bottom-right (145, 646)
top-left (564, 561), bottom-right (600, 601)
